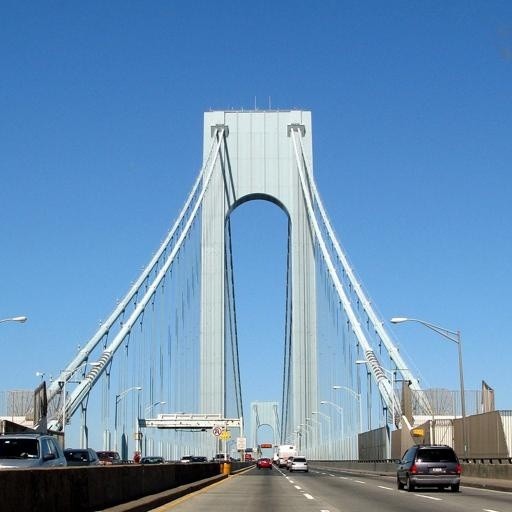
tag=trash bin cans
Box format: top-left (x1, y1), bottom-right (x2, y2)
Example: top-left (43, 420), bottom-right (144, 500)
top-left (223, 462), bottom-right (231, 475)
top-left (220, 463), bottom-right (224, 474)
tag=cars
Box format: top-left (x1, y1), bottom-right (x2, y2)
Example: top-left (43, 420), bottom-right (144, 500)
top-left (257, 458), bottom-right (272, 469)
top-left (63, 448), bottom-right (100, 465)
top-left (286, 456), bottom-right (308, 473)
top-left (140, 456), bottom-right (166, 465)
top-left (181, 452), bottom-right (225, 463)
top-left (96, 451), bottom-right (121, 465)
top-left (396, 444), bottom-right (461, 491)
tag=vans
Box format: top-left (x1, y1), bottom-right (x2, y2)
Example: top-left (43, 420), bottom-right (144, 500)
top-left (0, 434), bottom-right (68, 469)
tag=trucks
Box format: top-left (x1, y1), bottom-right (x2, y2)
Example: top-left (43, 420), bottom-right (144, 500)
top-left (274, 445), bottom-right (297, 468)
top-left (245, 454), bottom-right (252, 460)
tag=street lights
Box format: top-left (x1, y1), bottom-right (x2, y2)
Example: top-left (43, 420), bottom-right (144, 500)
top-left (296, 402), bottom-right (343, 460)
top-left (356, 360), bottom-right (396, 429)
top-left (334, 385), bottom-right (361, 432)
top-left (1, 316), bottom-right (26, 325)
top-left (62, 363), bottom-right (101, 434)
top-left (392, 318), bottom-right (468, 462)
top-left (114, 387), bottom-right (142, 450)
top-left (144, 401), bottom-right (165, 456)
